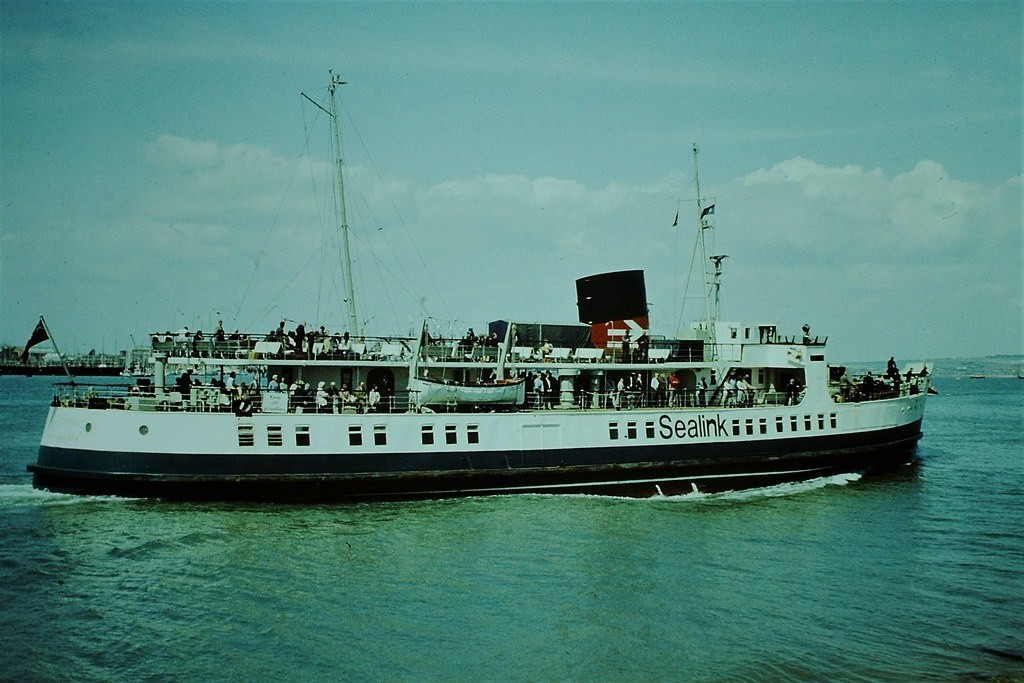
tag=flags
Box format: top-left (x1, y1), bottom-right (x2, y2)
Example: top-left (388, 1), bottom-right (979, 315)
top-left (672, 212), bottom-right (678, 227)
top-left (700, 204), bottom-right (714, 220)
top-left (20, 322), bottom-right (48, 365)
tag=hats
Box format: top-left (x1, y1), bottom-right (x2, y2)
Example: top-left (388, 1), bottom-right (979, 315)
top-left (330, 382), bottom-right (336, 386)
top-left (655, 372), bottom-right (659, 375)
top-left (296, 380), bottom-right (305, 384)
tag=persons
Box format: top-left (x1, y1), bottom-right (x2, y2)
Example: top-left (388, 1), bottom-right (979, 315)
top-left (50, 319), bottom-right (929, 416)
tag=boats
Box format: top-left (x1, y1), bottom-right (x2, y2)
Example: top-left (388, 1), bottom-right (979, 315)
top-left (24, 64), bottom-right (941, 501)
